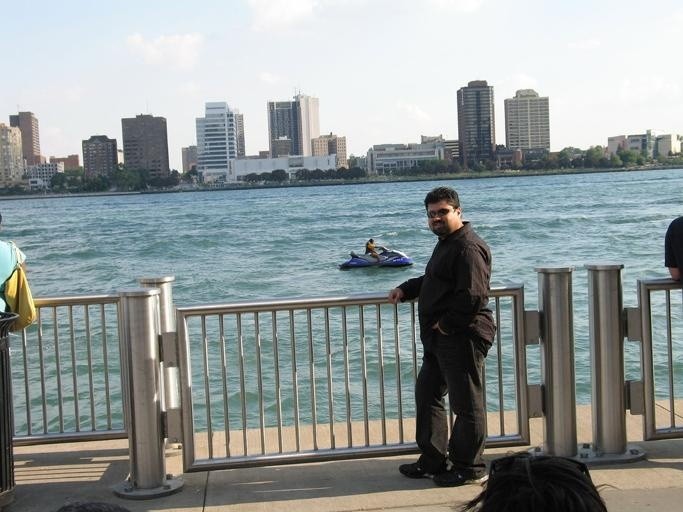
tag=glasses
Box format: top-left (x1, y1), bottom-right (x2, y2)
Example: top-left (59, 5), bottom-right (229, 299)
top-left (426, 207), bottom-right (455, 219)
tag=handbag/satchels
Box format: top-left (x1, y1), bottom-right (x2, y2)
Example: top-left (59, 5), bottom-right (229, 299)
top-left (2, 239), bottom-right (37, 332)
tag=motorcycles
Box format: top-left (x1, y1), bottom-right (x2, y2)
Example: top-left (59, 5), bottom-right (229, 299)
top-left (339, 246), bottom-right (413, 268)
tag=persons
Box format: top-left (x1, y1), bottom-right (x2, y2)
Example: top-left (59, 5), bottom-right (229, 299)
top-left (389, 185), bottom-right (496, 488)
top-left (0, 215), bottom-right (38, 335)
top-left (364, 238), bottom-right (384, 264)
top-left (662, 215), bottom-right (683, 297)
top-left (457, 449), bottom-right (611, 512)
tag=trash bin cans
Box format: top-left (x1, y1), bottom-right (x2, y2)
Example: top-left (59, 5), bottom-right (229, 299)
top-left (0, 312), bottom-right (20, 507)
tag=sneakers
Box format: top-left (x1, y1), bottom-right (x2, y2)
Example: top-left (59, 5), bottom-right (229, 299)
top-left (399, 460), bottom-right (451, 480)
top-left (433, 465), bottom-right (489, 488)
